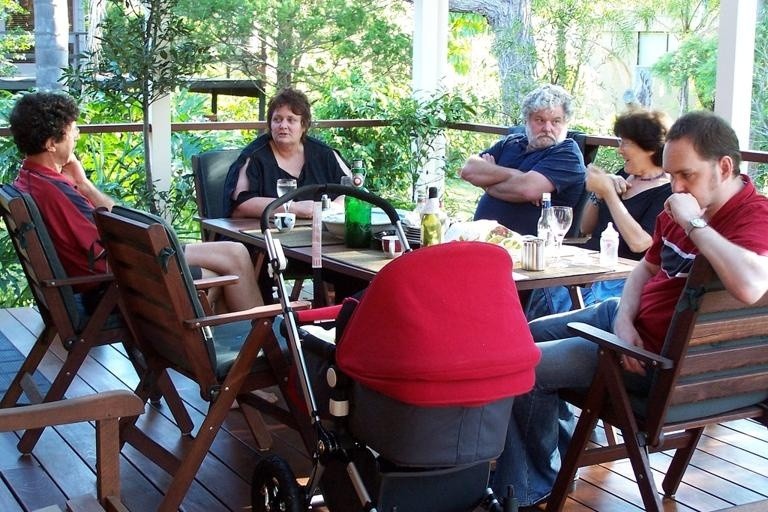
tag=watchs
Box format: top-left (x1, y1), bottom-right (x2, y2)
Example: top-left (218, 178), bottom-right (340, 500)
top-left (684, 218), bottom-right (707, 237)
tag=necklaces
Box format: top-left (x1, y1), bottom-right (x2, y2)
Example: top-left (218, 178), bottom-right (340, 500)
top-left (635, 171), bottom-right (670, 184)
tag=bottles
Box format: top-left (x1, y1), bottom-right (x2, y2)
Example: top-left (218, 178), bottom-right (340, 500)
top-left (344, 159), bottom-right (371, 248)
top-left (536, 192), bottom-right (560, 269)
top-left (600, 222), bottom-right (620, 266)
top-left (420, 188), bottom-right (442, 248)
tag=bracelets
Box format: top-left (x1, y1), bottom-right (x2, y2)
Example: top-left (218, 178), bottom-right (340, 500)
top-left (587, 190), bottom-right (602, 206)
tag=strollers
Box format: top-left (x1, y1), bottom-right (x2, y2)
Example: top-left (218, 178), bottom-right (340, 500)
top-left (250, 182), bottom-right (543, 512)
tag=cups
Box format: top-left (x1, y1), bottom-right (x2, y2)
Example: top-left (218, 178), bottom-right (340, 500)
top-left (274, 212), bottom-right (296, 233)
top-left (381, 236), bottom-right (405, 259)
top-left (521, 238), bottom-right (545, 272)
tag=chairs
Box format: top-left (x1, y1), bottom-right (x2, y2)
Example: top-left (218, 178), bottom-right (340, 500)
top-left (545, 254), bottom-right (768, 512)
top-left (0, 389), bottom-right (144, 512)
top-left (90, 203), bottom-right (317, 511)
top-left (191, 150), bottom-right (246, 241)
top-left (0, 185), bottom-right (239, 455)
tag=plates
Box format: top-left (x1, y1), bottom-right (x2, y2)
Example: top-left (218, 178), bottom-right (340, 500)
top-left (405, 213), bottom-right (420, 245)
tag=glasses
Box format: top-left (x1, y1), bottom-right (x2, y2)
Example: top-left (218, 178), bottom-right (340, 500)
top-left (616, 138), bottom-right (635, 147)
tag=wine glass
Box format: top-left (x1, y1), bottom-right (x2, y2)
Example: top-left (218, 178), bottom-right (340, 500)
top-left (546, 206), bottom-right (574, 270)
top-left (276, 179), bottom-right (297, 213)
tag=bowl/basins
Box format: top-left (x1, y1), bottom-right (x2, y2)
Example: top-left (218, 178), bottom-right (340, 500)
top-left (322, 207), bottom-right (401, 241)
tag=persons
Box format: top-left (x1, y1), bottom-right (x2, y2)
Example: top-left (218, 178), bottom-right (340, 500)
top-left (225, 87), bottom-right (356, 219)
top-left (461, 83), bottom-right (587, 239)
top-left (524, 107), bottom-right (675, 322)
top-left (10, 93), bottom-right (264, 317)
top-left (492, 109), bottom-right (768, 511)
